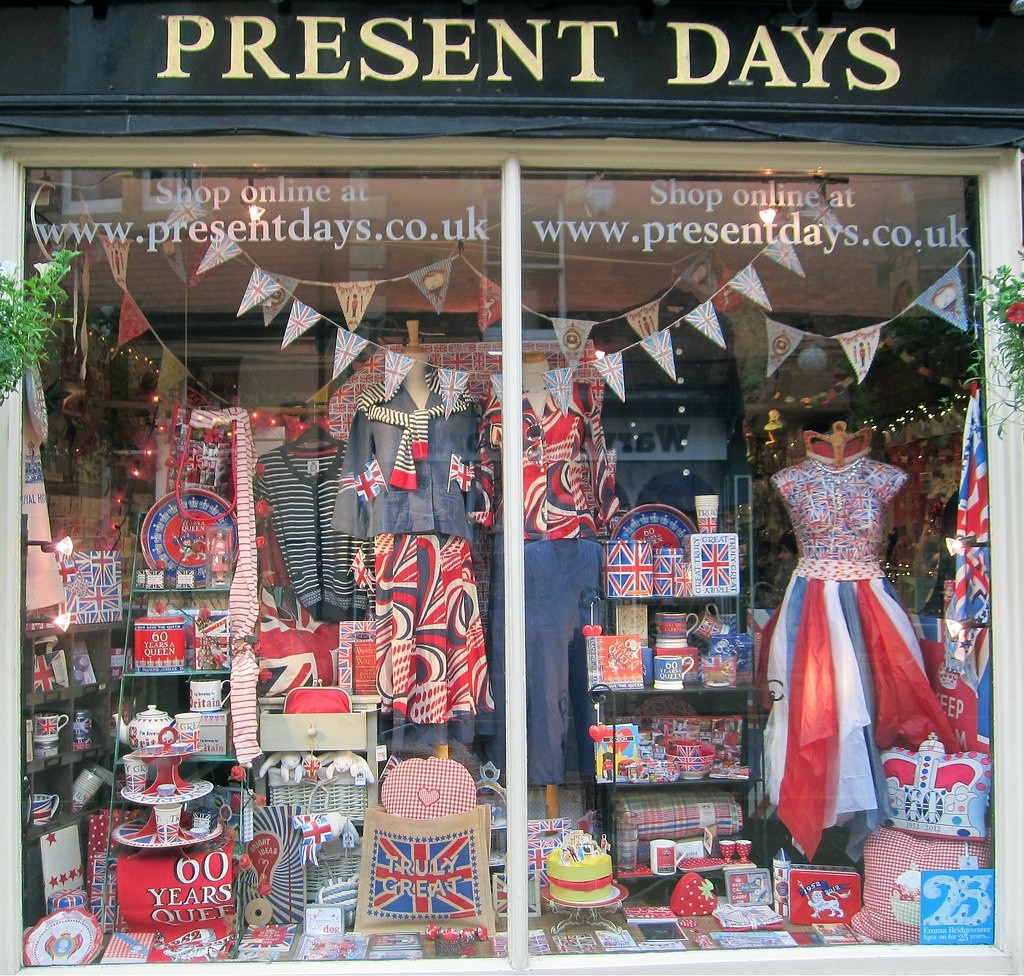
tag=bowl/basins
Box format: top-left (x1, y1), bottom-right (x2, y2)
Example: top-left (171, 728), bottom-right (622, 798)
top-left (157, 784), bottom-right (176, 796)
top-left (147, 743), bottom-right (190, 754)
top-left (190, 827), bottom-right (207, 839)
top-left (667, 749), bottom-right (716, 780)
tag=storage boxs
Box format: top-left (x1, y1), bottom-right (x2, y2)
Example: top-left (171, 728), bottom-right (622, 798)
top-left (191, 709), bottom-right (234, 754)
top-left (135, 569), bottom-right (196, 590)
top-left (587, 533), bottom-right (753, 784)
top-left (788, 863), bottom-right (861, 924)
top-left (266, 766), bottom-right (369, 824)
top-left (133, 616), bottom-right (187, 672)
top-left (49, 889), bottom-right (89, 915)
top-left (302, 839), bottom-right (364, 902)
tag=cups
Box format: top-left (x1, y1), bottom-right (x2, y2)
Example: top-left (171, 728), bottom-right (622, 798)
top-left (33, 712), bottom-right (69, 741)
top-left (650, 839), bottom-right (687, 876)
top-left (123, 754), bottom-right (148, 793)
top-left (73, 768), bottom-right (102, 813)
top-left (33, 793), bottom-right (60, 826)
top-left (675, 745), bottom-right (703, 758)
top-left (154, 804), bottom-right (183, 843)
top-left (175, 713), bottom-right (201, 751)
top-left (691, 603), bottom-right (730, 640)
top-left (655, 612), bottom-right (699, 648)
top-left (695, 494), bottom-right (720, 533)
top-left (654, 655), bottom-right (695, 689)
top-left (189, 679), bottom-right (233, 712)
top-left (615, 811), bottom-right (640, 871)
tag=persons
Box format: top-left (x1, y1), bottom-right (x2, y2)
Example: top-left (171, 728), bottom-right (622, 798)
top-left (480, 352), bottom-right (617, 785)
top-left (332, 348), bottom-right (495, 720)
top-left (757, 429), bottom-right (957, 864)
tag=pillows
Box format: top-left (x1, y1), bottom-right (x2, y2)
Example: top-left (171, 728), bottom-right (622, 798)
top-left (879, 732), bottom-right (992, 842)
top-left (851, 826), bottom-right (991, 944)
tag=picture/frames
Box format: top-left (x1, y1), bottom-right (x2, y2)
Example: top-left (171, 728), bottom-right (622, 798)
top-left (725, 867), bottom-right (773, 907)
top-left (301, 903), bottom-right (346, 937)
top-left (491, 871), bottom-right (542, 918)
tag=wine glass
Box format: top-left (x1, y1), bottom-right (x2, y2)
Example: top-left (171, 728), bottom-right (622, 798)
top-left (718, 840), bottom-right (735, 864)
top-left (736, 840), bottom-right (752, 864)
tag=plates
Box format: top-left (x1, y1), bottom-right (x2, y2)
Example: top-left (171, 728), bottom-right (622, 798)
top-left (23, 908), bottom-right (102, 965)
top-left (538, 882), bottom-right (630, 908)
top-left (140, 489), bottom-right (239, 589)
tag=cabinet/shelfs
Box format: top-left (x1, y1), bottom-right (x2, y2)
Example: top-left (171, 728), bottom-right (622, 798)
top-left (25, 619), bottom-right (139, 933)
top-left (584, 497), bottom-right (764, 900)
top-left (102, 504), bottom-right (257, 956)
top-left (258, 694), bottom-right (380, 925)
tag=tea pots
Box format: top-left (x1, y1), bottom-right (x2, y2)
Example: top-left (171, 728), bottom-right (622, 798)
top-left (112, 704), bottom-right (176, 750)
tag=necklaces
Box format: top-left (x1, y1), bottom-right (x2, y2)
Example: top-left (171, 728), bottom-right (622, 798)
top-left (811, 458), bottom-right (866, 509)
